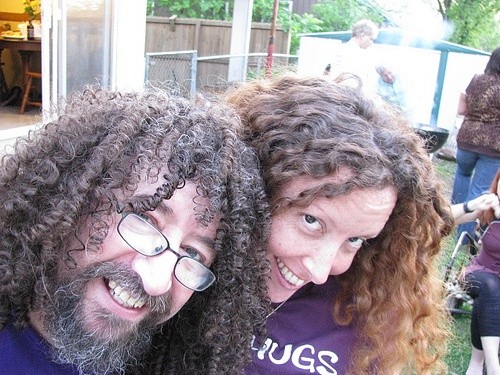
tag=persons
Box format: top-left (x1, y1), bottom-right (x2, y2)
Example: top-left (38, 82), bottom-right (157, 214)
top-left (321, 17), bottom-right (415, 117)
top-left (452, 47), bottom-right (500, 245)
top-left (233, 64), bottom-right (459, 375)
top-left (448, 168), bottom-right (500, 375)
top-left (0, 79), bottom-right (272, 375)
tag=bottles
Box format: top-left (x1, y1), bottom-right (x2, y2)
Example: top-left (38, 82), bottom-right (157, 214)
top-left (27, 20), bottom-right (34, 40)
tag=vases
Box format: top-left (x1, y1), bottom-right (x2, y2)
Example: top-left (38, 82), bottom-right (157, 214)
top-left (26, 21), bottom-right (35, 39)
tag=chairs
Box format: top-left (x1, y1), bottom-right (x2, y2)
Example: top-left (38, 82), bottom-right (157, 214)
top-left (18, 49), bottom-right (41, 113)
top-left (441, 221), bottom-right (488, 318)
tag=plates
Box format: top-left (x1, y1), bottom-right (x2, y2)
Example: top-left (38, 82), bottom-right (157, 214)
top-left (2, 36), bottom-right (24, 40)
top-left (33, 36), bottom-right (41, 40)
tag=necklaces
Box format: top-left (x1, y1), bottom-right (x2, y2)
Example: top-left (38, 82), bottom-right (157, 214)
top-left (252, 300), bottom-right (286, 328)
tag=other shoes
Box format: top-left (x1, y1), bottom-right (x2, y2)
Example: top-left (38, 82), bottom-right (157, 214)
top-left (0, 86), bottom-right (20, 107)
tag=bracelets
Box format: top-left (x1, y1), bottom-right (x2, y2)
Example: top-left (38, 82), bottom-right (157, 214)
top-left (463, 201), bottom-right (474, 213)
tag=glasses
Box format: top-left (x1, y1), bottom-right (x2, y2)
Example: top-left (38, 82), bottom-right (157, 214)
top-left (117, 209), bottom-right (216, 292)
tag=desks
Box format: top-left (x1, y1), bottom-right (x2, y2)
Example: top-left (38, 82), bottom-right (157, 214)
top-left (0, 29), bottom-right (42, 109)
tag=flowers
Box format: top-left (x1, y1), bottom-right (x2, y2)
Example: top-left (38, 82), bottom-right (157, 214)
top-left (22, 0), bottom-right (40, 22)
